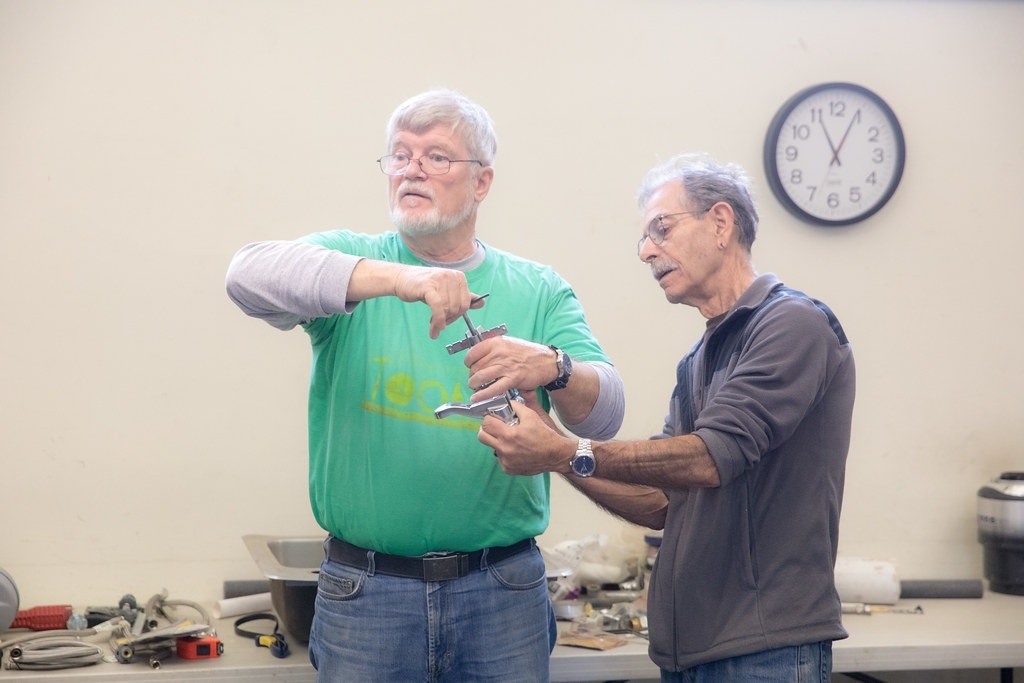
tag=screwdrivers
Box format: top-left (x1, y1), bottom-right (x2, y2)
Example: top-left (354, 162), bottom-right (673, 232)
top-left (119, 593), bottom-right (136, 612)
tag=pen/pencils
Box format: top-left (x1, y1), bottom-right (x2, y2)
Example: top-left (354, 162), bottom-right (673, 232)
top-left (867, 606), bottom-right (924, 613)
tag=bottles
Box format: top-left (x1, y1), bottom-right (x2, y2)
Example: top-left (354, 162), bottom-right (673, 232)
top-left (636, 534), bottom-right (663, 639)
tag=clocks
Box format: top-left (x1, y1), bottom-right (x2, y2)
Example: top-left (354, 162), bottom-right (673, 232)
top-left (762, 81), bottom-right (906, 226)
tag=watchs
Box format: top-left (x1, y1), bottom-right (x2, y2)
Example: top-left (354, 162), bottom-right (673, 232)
top-left (544, 345), bottom-right (572, 391)
top-left (570, 438), bottom-right (596, 477)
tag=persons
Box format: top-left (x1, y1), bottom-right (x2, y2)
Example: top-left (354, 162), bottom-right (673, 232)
top-left (478, 166), bottom-right (855, 683)
top-left (226, 92), bottom-right (625, 683)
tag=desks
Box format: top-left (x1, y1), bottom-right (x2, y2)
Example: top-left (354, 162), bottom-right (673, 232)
top-left (0, 589), bottom-right (1024, 683)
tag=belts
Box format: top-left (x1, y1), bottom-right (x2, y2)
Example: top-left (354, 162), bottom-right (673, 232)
top-left (328, 537), bottom-right (532, 582)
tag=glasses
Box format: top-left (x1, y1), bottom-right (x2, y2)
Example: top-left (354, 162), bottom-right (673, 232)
top-left (378, 154), bottom-right (483, 176)
top-left (637, 208), bottom-right (709, 264)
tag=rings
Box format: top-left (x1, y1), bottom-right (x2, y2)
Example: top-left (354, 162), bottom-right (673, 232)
top-left (494, 450), bottom-right (497, 457)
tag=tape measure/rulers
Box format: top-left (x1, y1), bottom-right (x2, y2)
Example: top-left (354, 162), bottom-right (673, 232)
top-left (175, 627), bottom-right (225, 659)
